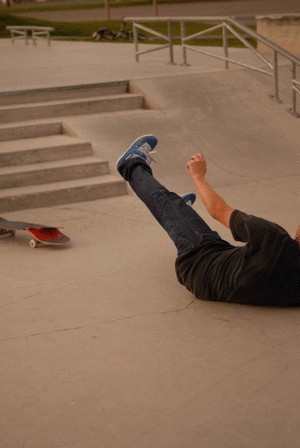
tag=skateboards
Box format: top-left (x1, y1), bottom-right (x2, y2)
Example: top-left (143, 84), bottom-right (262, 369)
top-left (0, 215), bottom-right (72, 250)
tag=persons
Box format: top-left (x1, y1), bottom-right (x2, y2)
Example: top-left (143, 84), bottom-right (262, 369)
top-left (115, 133), bottom-right (300, 307)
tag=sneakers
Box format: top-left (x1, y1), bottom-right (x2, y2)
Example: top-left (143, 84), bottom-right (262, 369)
top-left (116, 134), bottom-right (157, 172)
top-left (181, 192), bottom-right (196, 206)
